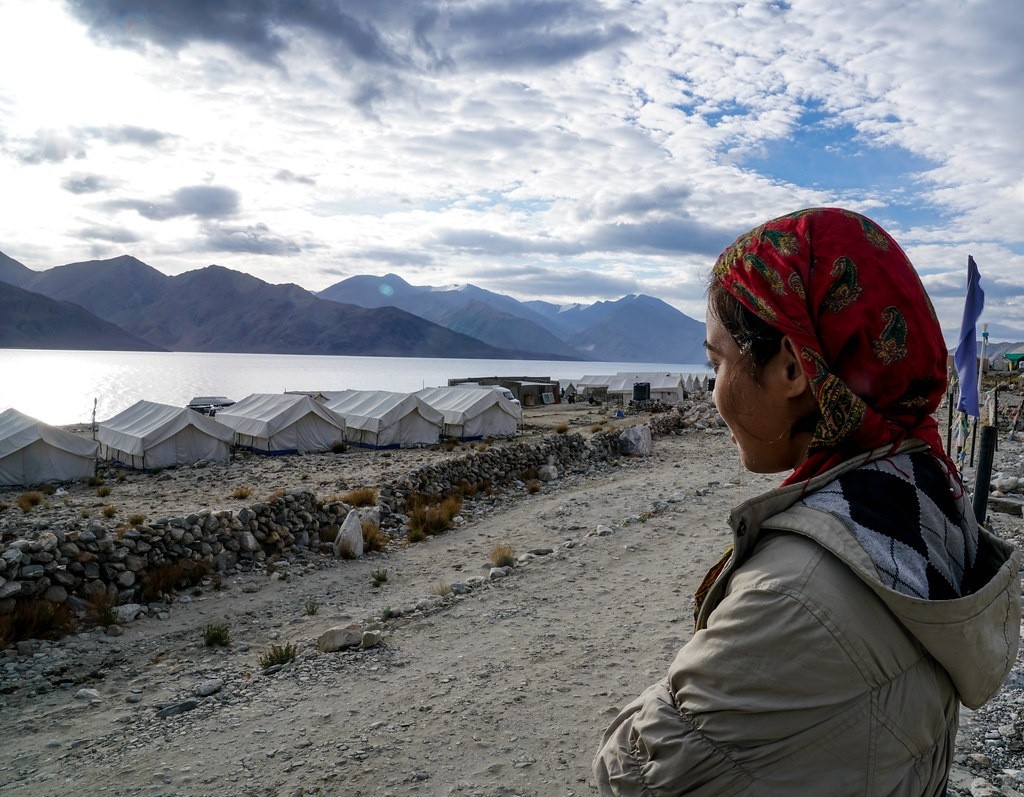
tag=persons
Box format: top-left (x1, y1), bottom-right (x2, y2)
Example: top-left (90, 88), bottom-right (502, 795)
top-left (592, 208), bottom-right (1020, 797)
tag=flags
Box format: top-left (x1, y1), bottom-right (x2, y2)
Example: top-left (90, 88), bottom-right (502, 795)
top-left (953, 254), bottom-right (985, 415)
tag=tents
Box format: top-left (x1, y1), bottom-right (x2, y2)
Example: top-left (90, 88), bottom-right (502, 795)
top-left (0, 408), bottom-right (98, 484)
top-left (98, 387), bottom-right (522, 471)
top-left (577, 371), bottom-right (708, 402)
